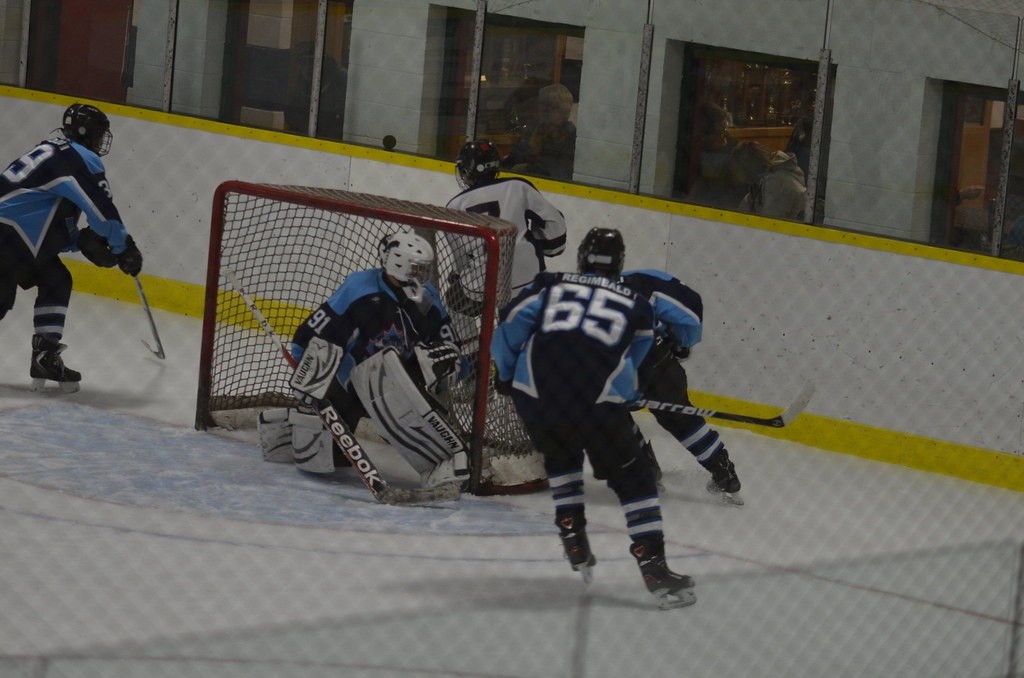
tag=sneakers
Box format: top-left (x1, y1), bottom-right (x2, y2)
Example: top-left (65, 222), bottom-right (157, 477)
top-left (641, 439), bottom-right (666, 492)
top-left (555, 511), bottom-right (596, 583)
top-left (30, 334), bottom-right (81, 393)
top-left (630, 532), bottom-right (697, 611)
top-left (705, 448), bottom-right (745, 505)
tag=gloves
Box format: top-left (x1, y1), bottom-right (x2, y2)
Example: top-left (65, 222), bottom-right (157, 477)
top-left (493, 371), bottom-right (512, 396)
top-left (76, 224), bottom-right (117, 268)
top-left (112, 234), bottom-right (143, 276)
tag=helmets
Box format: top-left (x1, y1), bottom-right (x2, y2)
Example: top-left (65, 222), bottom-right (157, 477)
top-left (378, 232), bottom-right (434, 303)
top-left (62, 103), bottom-right (113, 157)
top-left (454, 140), bottom-right (501, 187)
top-left (577, 226), bottom-right (625, 277)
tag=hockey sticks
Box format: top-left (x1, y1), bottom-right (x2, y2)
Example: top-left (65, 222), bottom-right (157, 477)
top-left (630, 379), bottom-right (818, 429)
top-left (135, 275), bottom-right (166, 360)
top-left (222, 267), bottom-right (461, 507)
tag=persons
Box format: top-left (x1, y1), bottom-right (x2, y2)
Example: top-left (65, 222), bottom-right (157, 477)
top-left (0, 102), bottom-right (143, 395)
top-left (678, 102), bottom-right (728, 179)
top-left (615, 268), bottom-right (744, 507)
top-left (488, 227), bottom-right (699, 609)
top-left (944, 185), bottom-right (986, 246)
top-left (787, 119), bottom-right (811, 177)
top-left (444, 138), bottom-right (567, 339)
top-left (726, 140), bottom-right (807, 224)
top-left (257, 232), bottom-right (491, 504)
top-left (504, 82), bottom-right (576, 172)
top-left (285, 40), bottom-right (344, 140)
top-left (511, 120), bottom-right (573, 181)
